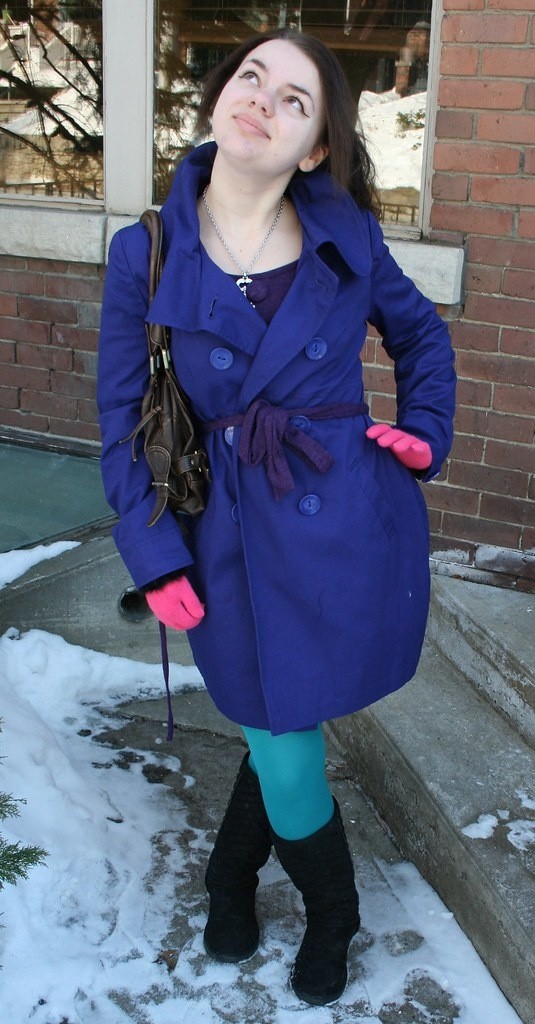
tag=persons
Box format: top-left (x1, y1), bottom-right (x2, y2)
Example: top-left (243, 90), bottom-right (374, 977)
top-left (96, 28), bottom-right (458, 1006)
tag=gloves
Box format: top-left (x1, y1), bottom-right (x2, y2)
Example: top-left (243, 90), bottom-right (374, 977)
top-left (367, 425), bottom-right (433, 469)
top-left (145, 575), bottom-right (206, 632)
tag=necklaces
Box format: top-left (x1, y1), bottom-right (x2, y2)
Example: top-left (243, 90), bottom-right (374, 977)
top-left (202, 185), bottom-right (284, 309)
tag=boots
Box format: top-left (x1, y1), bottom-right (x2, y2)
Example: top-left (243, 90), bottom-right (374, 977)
top-left (203, 751), bottom-right (275, 963)
top-left (268, 796), bottom-right (359, 1007)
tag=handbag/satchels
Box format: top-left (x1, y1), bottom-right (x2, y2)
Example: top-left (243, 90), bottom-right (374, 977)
top-left (117, 209), bottom-right (211, 528)
